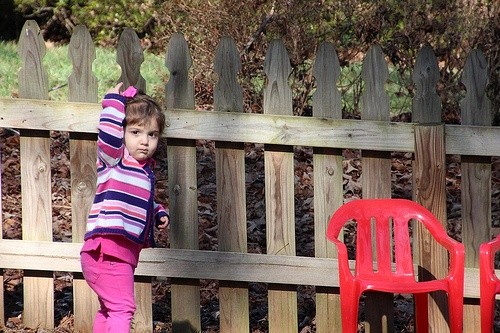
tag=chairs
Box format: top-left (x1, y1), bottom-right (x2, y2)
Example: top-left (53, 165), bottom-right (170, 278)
top-left (477, 232), bottom-right (500, 333)
top-left (325, 198), bottom-right (465, 332)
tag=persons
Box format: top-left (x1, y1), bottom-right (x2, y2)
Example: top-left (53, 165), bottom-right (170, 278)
top-left (79, 81), bottom-right (170, 333)
